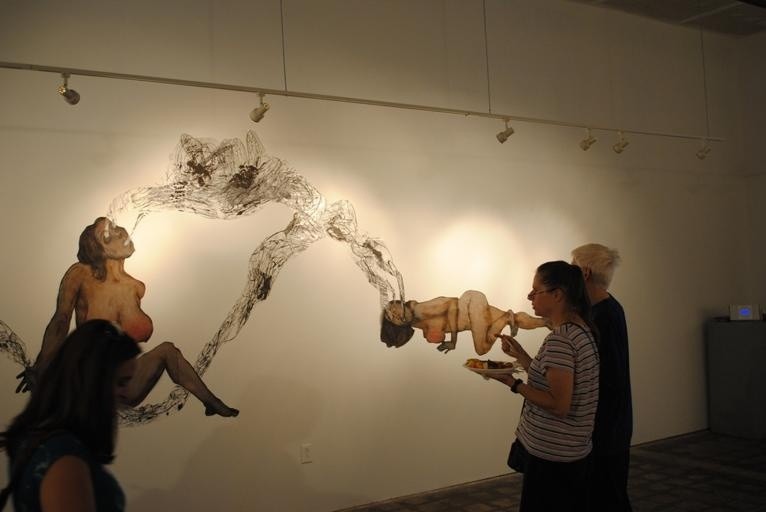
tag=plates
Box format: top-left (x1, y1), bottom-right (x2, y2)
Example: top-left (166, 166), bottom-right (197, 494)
top-left (462, 360), bottom-right (514, 376)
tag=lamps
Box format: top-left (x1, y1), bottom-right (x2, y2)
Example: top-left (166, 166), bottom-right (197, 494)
top-left (250, 92), bottom-right (271, 122)
top-left (496, 119), bottom-right (515, 143)
top-left (58, 60), bottom-right (81, 106)
top-left (579, 128), bottom-right (630, 154)
top-left (697, 140), bottom-right (712, 159)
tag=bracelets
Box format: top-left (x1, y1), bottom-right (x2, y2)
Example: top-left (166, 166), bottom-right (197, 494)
top-left (510, 378), bottom-right (523, 393)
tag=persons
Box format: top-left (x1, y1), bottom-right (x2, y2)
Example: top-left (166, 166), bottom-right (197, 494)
top-left (378, 287), bottom-right (550, 357)
top-left (568, 242), bottom-right (634, 510)
top-left (482, 260), bottom-right (605, 510)
top-left (13, 214), bottom-right (243, 421)
top-left (3, 316), bottom-right (145, 510)
